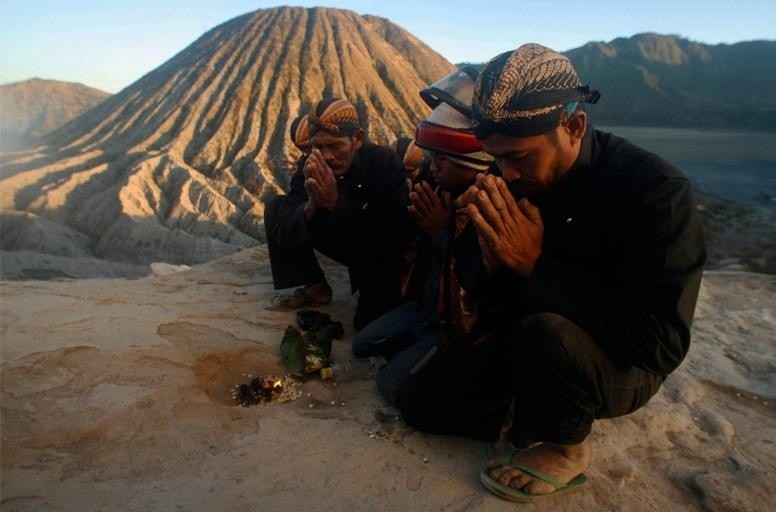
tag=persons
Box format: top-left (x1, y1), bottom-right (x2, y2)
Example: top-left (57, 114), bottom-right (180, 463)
top-left (353, 63), bottom-right (502, 403)
top-left (401, 45), bottom-right (708, 502)
top-left (393, 139), bottom-right (425, 179)
top-left (262, 99), bottom-right (412, 330)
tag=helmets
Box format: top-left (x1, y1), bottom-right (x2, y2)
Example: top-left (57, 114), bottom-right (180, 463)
top-left (413, 66), bottom-right (486, 153)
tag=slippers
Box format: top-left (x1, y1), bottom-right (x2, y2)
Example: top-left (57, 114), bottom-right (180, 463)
top-left (479, 447), bottom-right (590, 504)
top-left (264, 288), bottom-right (332, 309)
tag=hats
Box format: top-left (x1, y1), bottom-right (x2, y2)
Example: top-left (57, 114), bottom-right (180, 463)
top-left (308, 97), bottom-right (358, 138)
top-left (389, 136), bottom-right (424, 173)
top-left (471, 44), bottom-right (587, 142)
top-left (290, 113), bottom-right (311, 153)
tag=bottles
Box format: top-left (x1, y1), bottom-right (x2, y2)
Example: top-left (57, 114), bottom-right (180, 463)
top-left (321, 356), bottom-right (387, 384)
